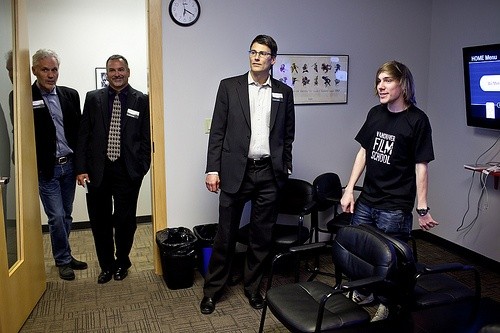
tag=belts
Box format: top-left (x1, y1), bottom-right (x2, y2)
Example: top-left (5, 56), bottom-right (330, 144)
top-left (248, 157), bottom-right (271, 165)
top-left (56, 155), bottom-right (73, 165)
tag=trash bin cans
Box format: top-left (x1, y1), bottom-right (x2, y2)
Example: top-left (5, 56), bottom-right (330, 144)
top-left (194, 223), bottom-right (241, 287)
top-left (154, 226), bottom-right (198, 291)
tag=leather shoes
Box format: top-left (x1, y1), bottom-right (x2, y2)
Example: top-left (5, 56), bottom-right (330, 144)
top-left (114, 267), bottom-right (127, 280)
top-left (98, 269), bottom-right (114, 283)
top-left (244, 290), bottom-right (264, 308)
top-left (199, 293), bottom-right (221, 313)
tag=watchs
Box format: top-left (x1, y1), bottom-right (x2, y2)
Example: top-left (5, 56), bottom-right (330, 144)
top-left (416, 207), bottom-right (430, 217)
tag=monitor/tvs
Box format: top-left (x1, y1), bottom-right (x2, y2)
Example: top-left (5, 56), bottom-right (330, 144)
top-left (462, 44), bottom-right (500, 130)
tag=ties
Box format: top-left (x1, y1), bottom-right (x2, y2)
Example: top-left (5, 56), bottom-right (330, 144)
top-left (106, 92), bottom-right (121, 162)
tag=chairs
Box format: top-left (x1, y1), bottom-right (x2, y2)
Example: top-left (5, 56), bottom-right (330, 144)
top-left (236, 173), bottom-right (482, 333)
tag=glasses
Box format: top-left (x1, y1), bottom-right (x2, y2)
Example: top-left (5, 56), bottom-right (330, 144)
top-left (249, 50), bottom-right (272, 58)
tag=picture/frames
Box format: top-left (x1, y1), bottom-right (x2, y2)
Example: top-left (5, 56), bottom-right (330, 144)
top-left (271, 54), bottom-right (349, 106)
top-left (95, 67), bottom-right (110, 90)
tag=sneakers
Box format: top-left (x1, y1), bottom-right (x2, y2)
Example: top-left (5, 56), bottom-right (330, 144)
top-left (346, 290), bottom-right (374, 305)
top-left (370, 303), bottom-right (389, 322)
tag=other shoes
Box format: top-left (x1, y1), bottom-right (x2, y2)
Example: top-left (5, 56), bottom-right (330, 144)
top-left (59, 265), bottom-right (74, 279)
top-left (55, 258), bottom-right (87, 270)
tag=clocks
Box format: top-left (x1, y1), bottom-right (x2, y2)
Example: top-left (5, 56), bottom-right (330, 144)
top-left (169, 0), bottom-right (201, 27)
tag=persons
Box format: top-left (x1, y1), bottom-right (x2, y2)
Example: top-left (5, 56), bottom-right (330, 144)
top-left (199, 34), bottom-right (295, 315)
top-left (76, 55), bottom-right (151, 284)
top-left (4, 51), bottom-right (14, 165)
top-left (31, 49), bottom-right (88, 280)
top-left (339, 61), bottom-right (441, 322)
